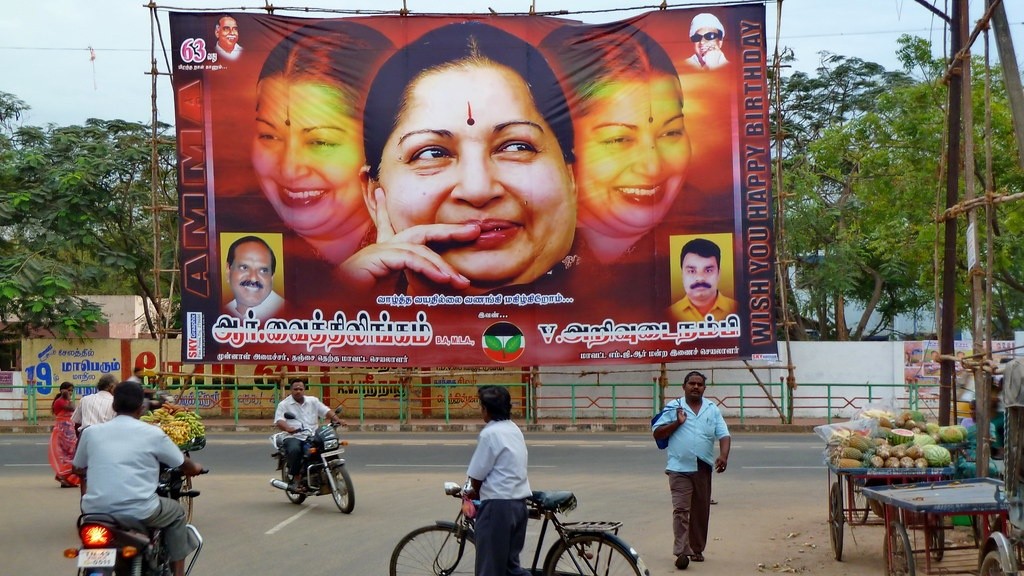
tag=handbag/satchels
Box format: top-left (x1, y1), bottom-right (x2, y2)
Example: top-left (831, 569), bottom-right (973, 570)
top-left (649, 397), bottom-right (682, 450)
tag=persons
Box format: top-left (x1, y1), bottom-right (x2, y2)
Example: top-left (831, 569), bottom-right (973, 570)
top-left (274, 379), bottom-right (346, 484)
top-left (652, 371), bottom-right (731, 568)
top-left (72, 381), bottom-right (201, 576)
top-left (959, 378), bottom-right (1006, 473)
top-left (225, 236), bottom-right (284, 320)
top-left (955, 351), bottom-right (965, 371)
top-left (70, 367), bottom-right (155, 512)
top-left (344, 21), bottom-right (578, 301)
top-left (670, 238), bottom-right (736, 322)
top-left (535, 24), bottom-right (692, 265)
top-left (215, 15), bottom-right (242, 61)
top-left (48, 382), bottom-right (81, 488)
top-left (685, 13), bottom-right (729, 70)
top-left (250, 22), bottom-right (398, 301)
top-left (468, 386), bottom-right (534, 576)
top-left (927, 351), bottom-right (940, 373)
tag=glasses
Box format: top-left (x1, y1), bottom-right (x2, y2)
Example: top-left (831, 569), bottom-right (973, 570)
top-left (690, 31), bottom-right (720, 43)
top-left (292, 388), bottom-right (306, 391)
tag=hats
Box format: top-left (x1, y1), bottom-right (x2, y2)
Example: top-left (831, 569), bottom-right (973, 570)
top-left (689, 13), bottom-right (725, 39)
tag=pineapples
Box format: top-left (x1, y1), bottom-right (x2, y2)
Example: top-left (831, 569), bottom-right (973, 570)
top-left (836, 433), bottom-right (877, 469)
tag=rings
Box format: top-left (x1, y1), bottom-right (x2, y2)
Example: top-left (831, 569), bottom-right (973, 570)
top-left (722, 467), bottom-right (724, 469)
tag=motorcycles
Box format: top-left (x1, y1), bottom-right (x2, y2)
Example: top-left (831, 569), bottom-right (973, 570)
top-left (269, 405), bottom-right (355, 514)
top-left (63, 436), bottom-right (209, 576)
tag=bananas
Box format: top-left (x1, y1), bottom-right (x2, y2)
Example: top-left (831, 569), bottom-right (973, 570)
top-left (140, 402), bottom-right (206, 446)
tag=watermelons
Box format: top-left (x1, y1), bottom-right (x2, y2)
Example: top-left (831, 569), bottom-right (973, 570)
top-left (887, 413), bottom-right (967, 467)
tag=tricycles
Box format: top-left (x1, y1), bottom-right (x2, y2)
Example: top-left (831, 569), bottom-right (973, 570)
top-left (825, 454), bottom-right (1024, 576)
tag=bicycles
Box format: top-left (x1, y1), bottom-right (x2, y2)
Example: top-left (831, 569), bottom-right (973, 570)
top-left (389, 476), bottom-right (650, 576)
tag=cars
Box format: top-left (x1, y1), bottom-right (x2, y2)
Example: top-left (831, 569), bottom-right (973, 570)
top-left (789, 250), bottom-right (973, 340)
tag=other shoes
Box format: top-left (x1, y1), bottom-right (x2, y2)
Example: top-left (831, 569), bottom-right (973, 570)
top-left (61, 483), bottom-right (79, 488)
top-left (55, 475), bottom-right (66, 483)
top-left (674, 553), bottom-right (689, 570)
top-left (690, 551), bottom-right (705, 562)
top-left (292, 476), bottom-right (305, 493)
